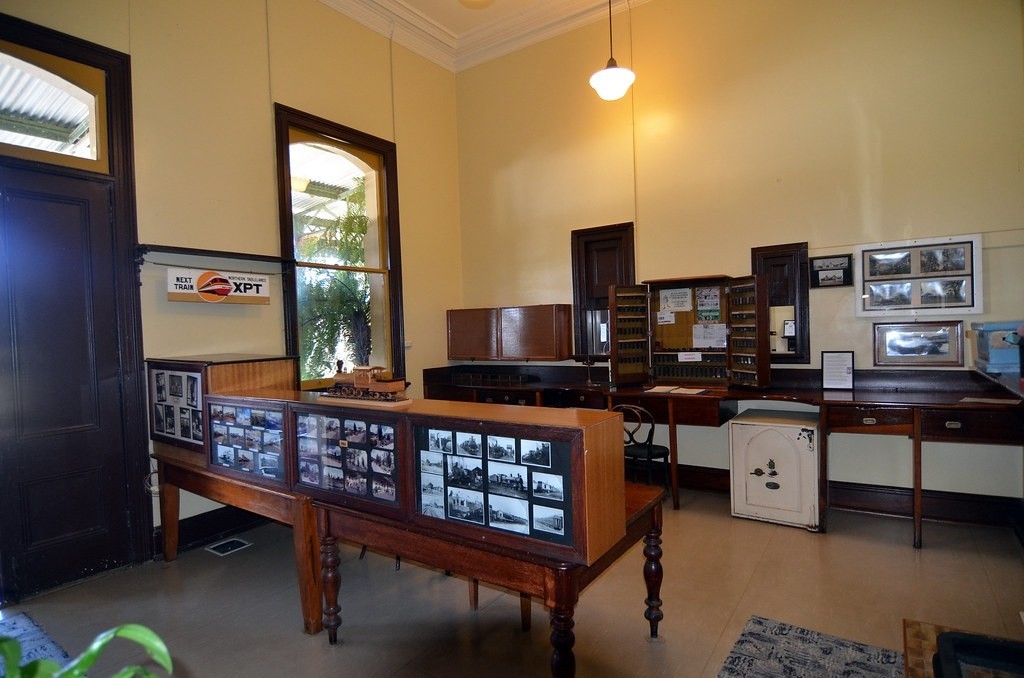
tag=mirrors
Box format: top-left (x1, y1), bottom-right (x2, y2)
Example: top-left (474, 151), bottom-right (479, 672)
top-left (586, 310), bottom-right (611, 355)
top-left (769, 306), bottom-right (796, 354)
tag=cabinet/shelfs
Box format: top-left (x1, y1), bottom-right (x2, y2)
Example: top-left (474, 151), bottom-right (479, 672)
top-left (447, 304), bottom-right (573, 362)
top-left (608, 274), bottom-right (771, 392)
top-left (202, 387), bottom-right (627, 568)
top-left (427, 378), bottom-right (1024, 549)
top-left (145, 352), bottom-right (301, 454)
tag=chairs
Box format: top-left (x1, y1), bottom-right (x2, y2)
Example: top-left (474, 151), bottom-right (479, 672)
top-left (612, 404), bottom-right (671, 498)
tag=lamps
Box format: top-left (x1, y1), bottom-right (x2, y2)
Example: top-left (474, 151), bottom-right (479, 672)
top-left (589, 0), bottom-right (635, 101)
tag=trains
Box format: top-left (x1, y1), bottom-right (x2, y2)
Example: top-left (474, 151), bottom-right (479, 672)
top-left (329, 360), bottom-right (410, 403)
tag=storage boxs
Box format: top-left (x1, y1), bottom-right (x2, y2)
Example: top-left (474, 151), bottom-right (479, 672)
top-left (973, 359), bottom-right (1021, 373)
top-left (971, 321), bottom-right (1024, 331)
top-left (977, 330), bottom-right (1024, 364)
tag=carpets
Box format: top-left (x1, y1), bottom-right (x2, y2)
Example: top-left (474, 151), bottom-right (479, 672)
top-left (903, 617), bottom-right (1024, 678)
top-left (0, 611), bottom-right (87, 678)
top-left (716, 615), bottom-right (905, 678)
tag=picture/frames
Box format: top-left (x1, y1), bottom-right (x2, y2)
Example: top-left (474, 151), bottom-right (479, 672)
top-left (854, 232), bottom-right (984, 318)
top-left (808, 253), bottom-right (854, 289)
top-left (871, 320), bottom-right (964, 367)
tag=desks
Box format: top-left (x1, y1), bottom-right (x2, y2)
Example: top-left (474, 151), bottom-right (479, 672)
top-left (309, 479), bottom-right (665, 678)
top-left (150, 446), bottom-right (323, 636)
